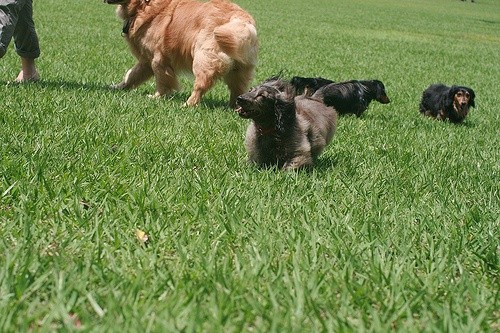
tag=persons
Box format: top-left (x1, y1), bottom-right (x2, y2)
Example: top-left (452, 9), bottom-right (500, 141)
top-left (0, 0), bottom-right (41, 83)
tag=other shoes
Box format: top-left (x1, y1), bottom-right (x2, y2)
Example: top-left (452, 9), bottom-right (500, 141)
top-left (15, 70), bottom-right (40, 82)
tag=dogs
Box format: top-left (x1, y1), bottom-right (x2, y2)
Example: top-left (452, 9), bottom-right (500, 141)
top-left (235, 68), bottom-right (339, 171)
top-left (419, 82), bottom-right (476, 123)
top-left (290, 76), bottom-right (335, 97)
top-left (312, 79), bottom-right (391, 118)
top-left (104, 0), bottom-right (258, 106)
